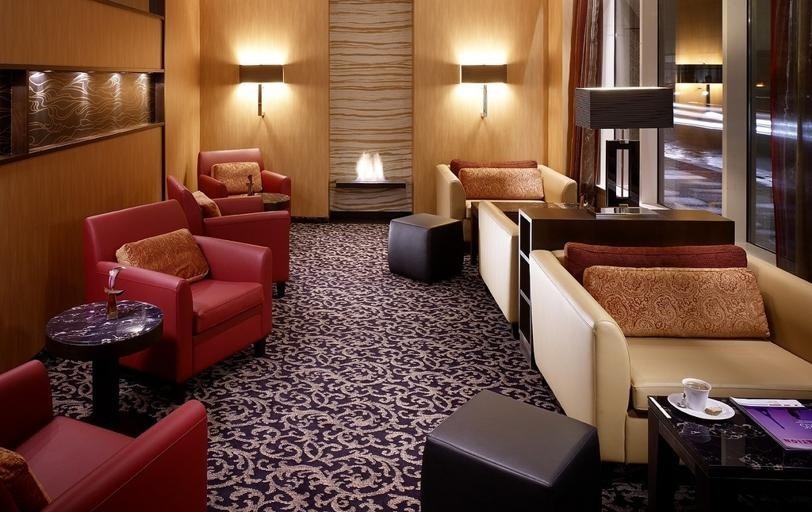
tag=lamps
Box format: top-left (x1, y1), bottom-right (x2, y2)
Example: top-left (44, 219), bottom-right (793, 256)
top-left (574, 87), bottom-right (673, 209)
top-left (678, 64), bottom-right (722, 106)
top-left (239, 65), bottom-right (283, 117)
top-left (462, 65), bottom-right (507, 118)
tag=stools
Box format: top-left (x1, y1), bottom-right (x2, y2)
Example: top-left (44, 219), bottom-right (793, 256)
top-left (388, 213), bottom-right (464, 283)
top-left (421, 389), bottom-right (603, 512)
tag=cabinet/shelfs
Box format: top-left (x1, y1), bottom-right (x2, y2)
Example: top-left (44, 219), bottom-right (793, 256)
top-left (517, 206), bottom-right (735, 366)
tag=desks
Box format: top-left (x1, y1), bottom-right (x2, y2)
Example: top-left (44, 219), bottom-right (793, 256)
top-left (647, 394), bottom-right (812, 511)
top-left (43, 298), bottom-right (163, 438)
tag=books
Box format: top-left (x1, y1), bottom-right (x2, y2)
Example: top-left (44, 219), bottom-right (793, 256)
top-left (730, 397), bottom-right (812, 451)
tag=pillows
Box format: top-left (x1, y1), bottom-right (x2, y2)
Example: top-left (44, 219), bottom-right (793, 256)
top-left (563, 242), bottom-right (771, 339)
top-left (116, 228), bottom-right (209, 284)
top-left (0, 446), bottom-right (52, 512)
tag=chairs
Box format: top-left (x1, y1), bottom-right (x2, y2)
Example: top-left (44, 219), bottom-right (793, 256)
top-left (436, 159), bottom-right (577, 338)
top-left (166, 148), bottom-right (291, 296)
top-left (84, 199), bottom-right (272, 404)
top-left (0, 359), bottom-right (208, 512)
top-left (529, 249), bottom-right (812, 486)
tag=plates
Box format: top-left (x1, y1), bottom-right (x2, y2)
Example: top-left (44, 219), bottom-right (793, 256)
top-left (667, 391), bottom-right (735, 420)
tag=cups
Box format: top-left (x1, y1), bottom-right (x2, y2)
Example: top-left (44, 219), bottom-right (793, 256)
top-left (682, 378), bottom-right (711, 411)
